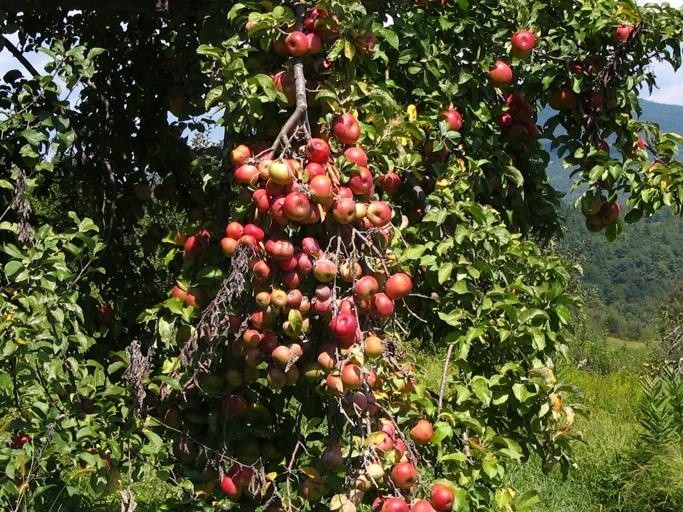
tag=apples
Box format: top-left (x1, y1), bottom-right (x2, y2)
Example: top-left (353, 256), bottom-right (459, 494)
top-left (137, 5), bottom-right (671, 511)
top-left (6, 434), bottom-right (30, 450)
top-left (79, 448), bottom-right (110, 472)
top-left (94, 304), bottom-right (112, 329)
